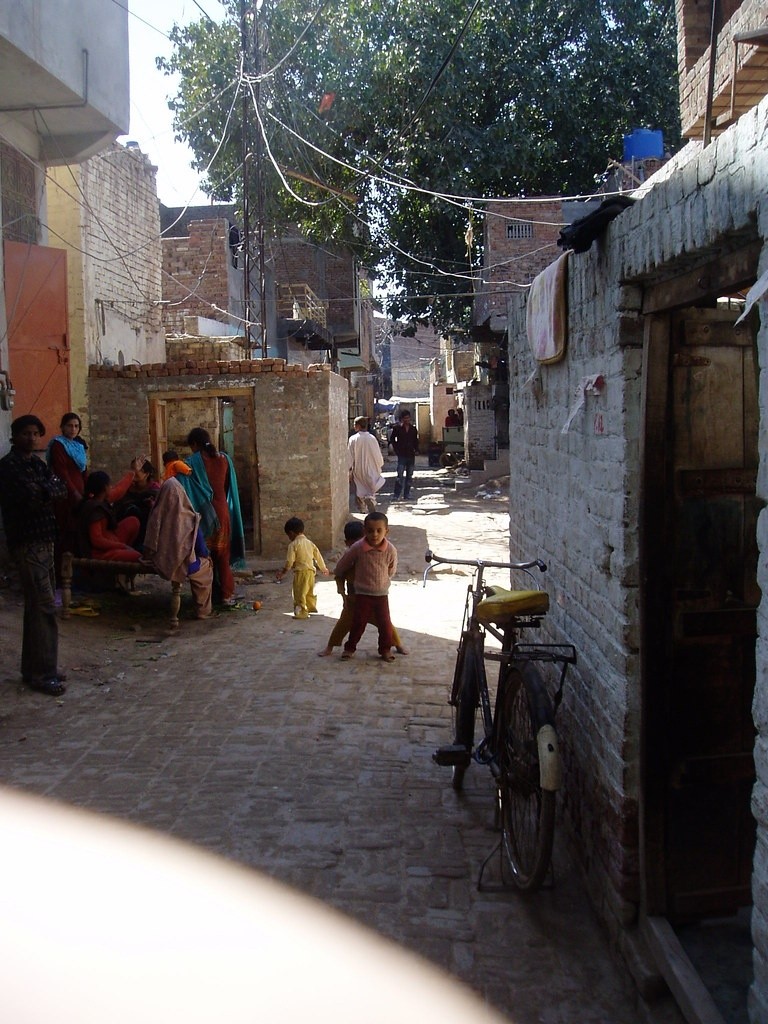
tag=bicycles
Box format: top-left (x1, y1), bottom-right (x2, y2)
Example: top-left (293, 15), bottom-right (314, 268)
top-left (420, 550), bottom-right (585, 898)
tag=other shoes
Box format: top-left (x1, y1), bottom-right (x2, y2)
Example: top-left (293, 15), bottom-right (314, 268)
top-left (359, 509), bottom-right (366, 514)
top-left (364, 498), bottom-right (375, 513)
top-left (22, 672), bottom-right (67, 696)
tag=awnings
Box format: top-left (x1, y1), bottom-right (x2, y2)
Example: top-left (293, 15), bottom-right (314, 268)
top-left (378, 395), bottom-right (430, 405)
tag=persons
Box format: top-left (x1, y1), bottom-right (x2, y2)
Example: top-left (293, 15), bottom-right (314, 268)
top-left (391, 410), bottom-right (419, 501)
top-left (317, 512), bottom-right (409, 661)
top-left (374, 413), bottom-right (395, 449)
top-left (0, 414), bottom-right (66, 695)
top-left (445, 408), bottom-right (464, 428)
top-left (45, 413), bottom-right (235, 627)
top-left (275, 516), bottom-right (329, 619)
top-left (347, 416), bottom-right (385, 515)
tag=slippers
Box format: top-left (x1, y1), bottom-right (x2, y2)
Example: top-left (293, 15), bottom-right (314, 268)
top-left (382, 653), bottom-right (397, 663)
top-left (200, 609), bottom-right (221, 619)
top-left (223, 600), bottom-right (236, 605)
top-left (70, 598), bottom-right (102, 616)
top-left (341, 651), bottom-right (352, 661)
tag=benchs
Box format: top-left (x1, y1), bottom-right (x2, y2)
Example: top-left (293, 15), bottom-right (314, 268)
top-left (60, 552), bottom-right (182, 629)
